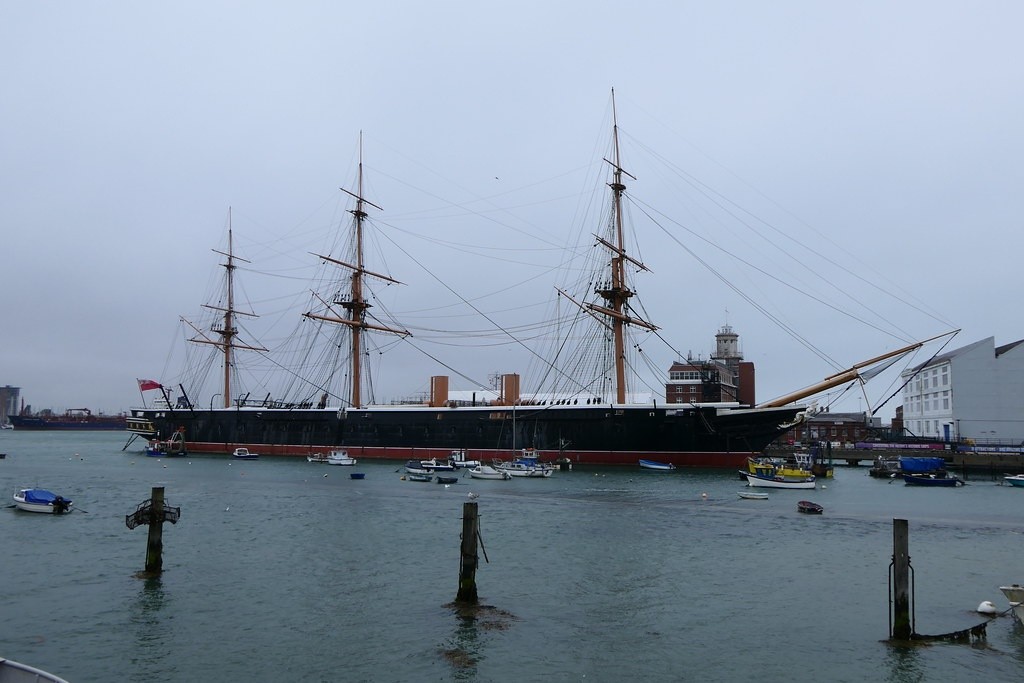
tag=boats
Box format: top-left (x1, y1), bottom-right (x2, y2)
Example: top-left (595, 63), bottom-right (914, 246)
top-left (233, 447), bottom-right (260, 459)
top-left (739, 453), bottom-right (833, 489)
top-left (306, 449), bottom-right (357, 465)
top-left (902, 473), bottom-right (967, 487)
top-left (870, 455), bottom-right (904, 479)
top-left (11, 489), bottom-right (74, 515)
top-left (143, 428), bottom-right (188, 458)
top-left (7, 398), bottom-right (126, 431)
top-left (638, 458), bottom-right (676, 469)
top-left (738, 491), bottom-right (768, 500)
top-left (798, 501), bottom-right (824, 515)
top-left (406, 447), bottom-right (559, 485)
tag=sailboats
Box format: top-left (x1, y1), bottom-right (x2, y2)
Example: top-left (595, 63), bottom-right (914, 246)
top-left (125, 84), bottom-right (811, 470)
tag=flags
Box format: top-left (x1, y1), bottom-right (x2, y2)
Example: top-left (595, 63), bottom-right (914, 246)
top-left (140, 379), bottom-right (161, 391)
